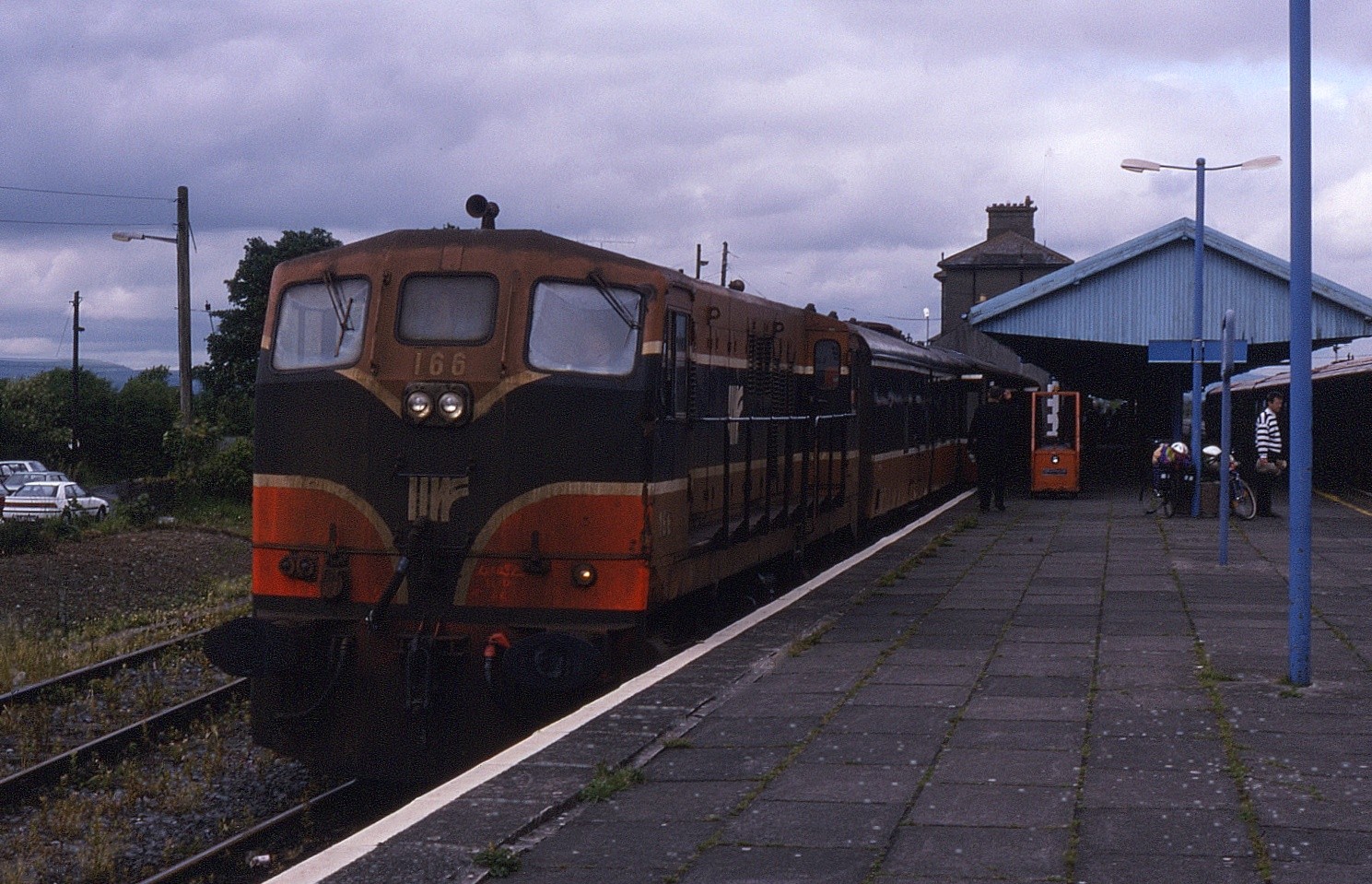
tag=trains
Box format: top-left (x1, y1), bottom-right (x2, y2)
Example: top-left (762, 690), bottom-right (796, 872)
top-left (250, 193), bottom-right (1042, 725)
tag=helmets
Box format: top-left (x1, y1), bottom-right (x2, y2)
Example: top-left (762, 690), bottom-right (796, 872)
top-left (1170, 442), bottom-right (1189, 458)
top-left (1202, 445), bottom-right (1223, 458)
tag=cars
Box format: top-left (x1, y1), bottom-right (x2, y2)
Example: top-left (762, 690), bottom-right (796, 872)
top-left (0, 461), bottom-right (110, 526)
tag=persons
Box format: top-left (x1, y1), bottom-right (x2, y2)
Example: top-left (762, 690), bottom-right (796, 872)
top-left (966, 386), bottom-right (1013, 514)
top-left (1254, 391), bottom-right (1284, 519)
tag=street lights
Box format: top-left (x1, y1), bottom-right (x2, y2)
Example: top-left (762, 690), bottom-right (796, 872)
top-left (1121, 153), bottom-right (1284, 518)
top-left (112, 228), bottom-right (193, 422)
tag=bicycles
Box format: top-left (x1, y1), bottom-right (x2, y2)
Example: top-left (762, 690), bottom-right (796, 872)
top-left (1139, 435), bottom-right (1194, 518)
top-left (1172, 446), bottom-right (1257, 521)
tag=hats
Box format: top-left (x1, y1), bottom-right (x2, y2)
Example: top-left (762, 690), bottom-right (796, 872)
top-left (987, 386), bottom-right (1007, 400)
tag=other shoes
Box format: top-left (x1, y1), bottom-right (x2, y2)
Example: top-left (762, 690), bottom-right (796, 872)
top-left (994, 498), bottom-right (1003, 507)
top-left (1256, 508), bottom-right (1276, 517)
top-left (979, 504), bottom-right (990, 514)
